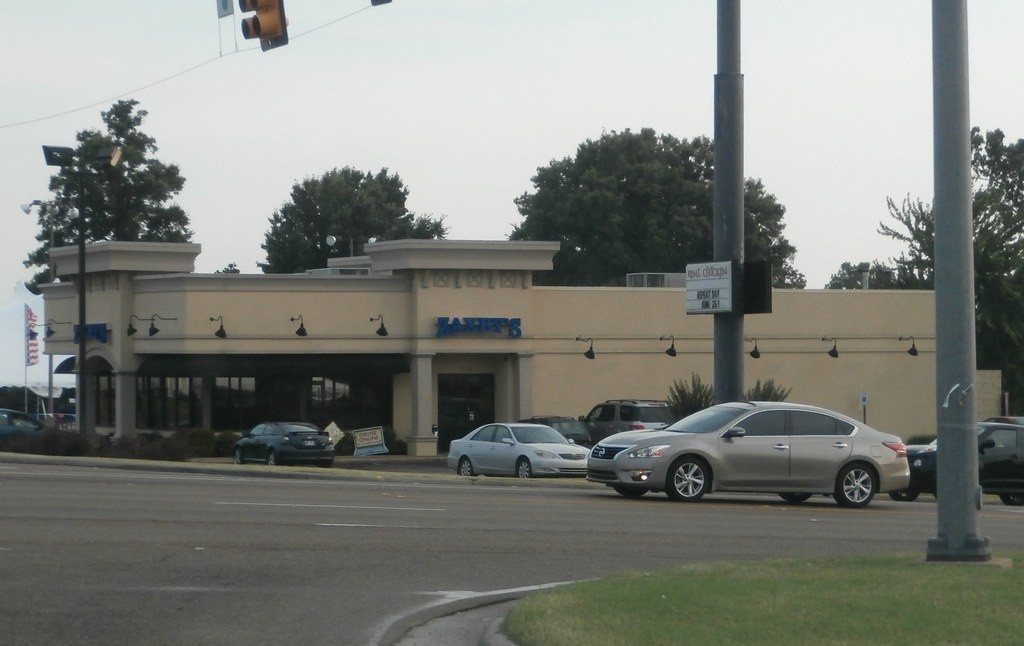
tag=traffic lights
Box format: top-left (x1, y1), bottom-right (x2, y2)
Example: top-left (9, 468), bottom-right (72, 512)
top-left (239, 0), bottom-right (290, 53)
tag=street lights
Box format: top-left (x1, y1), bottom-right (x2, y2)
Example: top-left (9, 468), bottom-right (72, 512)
top-left (43, 140), bottom-right (123, 446)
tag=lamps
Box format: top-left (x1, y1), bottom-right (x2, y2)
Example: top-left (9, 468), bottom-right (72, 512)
top-left (47, 319), bottom-right (71, 338)
top-left (291, 315), bottom-right (307, 336)
top-left (821, 337), bottom-right (839, 358)
top-left (576, 337), bottom-right (595, 360)
top-left (29, 322), bottom-right (53, 340)
top-left (210, 316), bottom-right (227, 338)
top-left (127, 314), bottom-right (155, 336)
top-left (659, 335), bottom-right (676, 356)
top-left (744, 337), bottom-right (761, 359)
top-left (149, 314), bottom-right (177, 336)
top-left (899, 336), bottom-right (918, 356)
top-left (370, 314), bottom-right (388, 336)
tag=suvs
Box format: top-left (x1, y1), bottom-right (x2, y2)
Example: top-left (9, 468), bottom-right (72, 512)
top-left (579, 397), bottom-right (678, 445)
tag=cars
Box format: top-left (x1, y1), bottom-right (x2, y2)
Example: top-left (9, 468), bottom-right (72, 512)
top-left (230, 421), bottom-right (336, 469)
top-left (583, 400), bottom-right (914, 507)
top-left (0, 408), bottom-right (60, 452)
top-left (518, 414), bottom-right (593, 450)
top-left (887, 415), bottom-right (1024, 506)
top-left (448, 423), bottom-right (594, 481)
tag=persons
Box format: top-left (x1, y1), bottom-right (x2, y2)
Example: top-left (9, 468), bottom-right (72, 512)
top-left (465, 393), bottom-right (479, 427)
top-left (990, 433), bottom-right (1004, 447)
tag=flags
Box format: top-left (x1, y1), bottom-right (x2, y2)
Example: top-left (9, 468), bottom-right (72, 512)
top-left (26, 304), bottom-right (39, 366)
